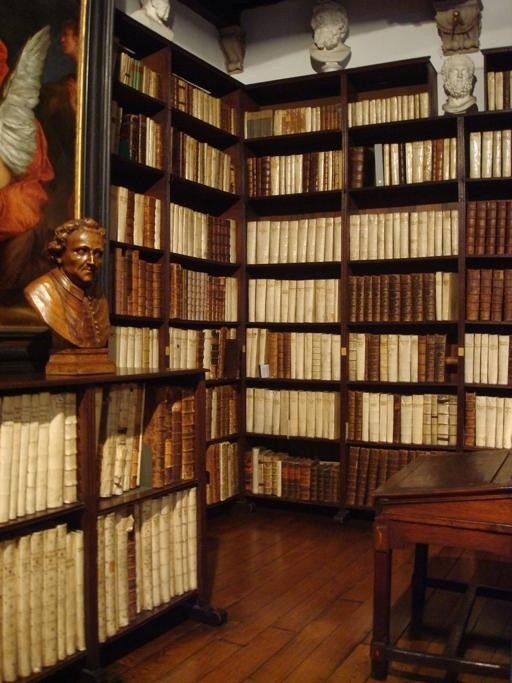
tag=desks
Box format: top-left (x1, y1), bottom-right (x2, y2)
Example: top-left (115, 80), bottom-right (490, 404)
top-left (371, 451), bottom-right (512, 679)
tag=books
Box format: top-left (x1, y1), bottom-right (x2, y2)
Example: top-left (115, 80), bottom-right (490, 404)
top-left (206, 382), bottom-right (241, 505)
top-left (348, 91), bottom-right (459, 262)
top-left (0, 380), bottom-right (198, 683)
top-left (109, 52), bottom-right (239, 379)
top-left (466, 131), bottom-right (511, 451)
top-left (346, 272), bottom-right (456, 507)
top-left (487, 71), bottom-right (511, 111)
top-left (244, 102), bottom-right (344, 504)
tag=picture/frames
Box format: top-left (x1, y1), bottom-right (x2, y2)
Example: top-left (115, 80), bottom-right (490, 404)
top-left (1, 0), bottom-right (92, 338)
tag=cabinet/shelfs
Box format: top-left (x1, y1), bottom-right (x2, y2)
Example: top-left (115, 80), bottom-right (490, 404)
top-left (244, 44), bottom-right (512, 515)
top-left (113, 11), bottom-right (244, 511)
top-left (0, 363), bottom-right (228, 680)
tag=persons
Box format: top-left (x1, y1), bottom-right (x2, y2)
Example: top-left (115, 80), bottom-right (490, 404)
top-left (23, 217), bottom-right (114, 348)
top-left (306, 3), bottom-right (355, 74)
top-left (442, 53), bottom-right (476, 113)
